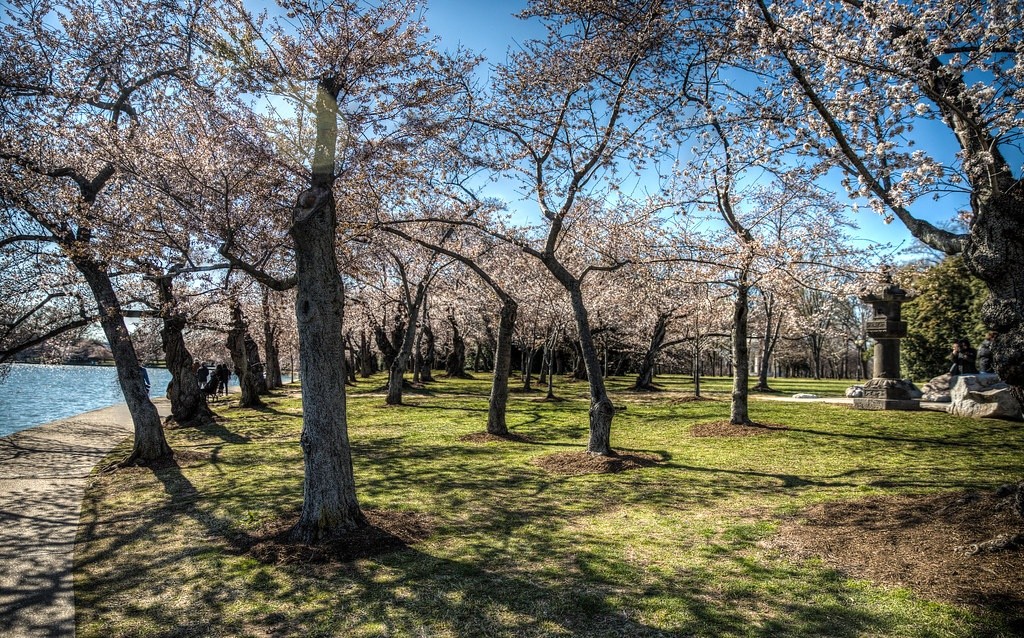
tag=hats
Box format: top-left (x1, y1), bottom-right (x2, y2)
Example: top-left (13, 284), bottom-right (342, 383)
top-left (201, 361), bottom-right (208, 365)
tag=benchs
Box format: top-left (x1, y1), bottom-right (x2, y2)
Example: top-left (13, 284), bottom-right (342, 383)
top-left (203, 382), bottom-right (219, 402)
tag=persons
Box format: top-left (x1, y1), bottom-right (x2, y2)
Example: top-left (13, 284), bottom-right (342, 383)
top-left (136, 359), bottom-right (151, 393)
top-left (193, 358), bottom-right (231, 397)
